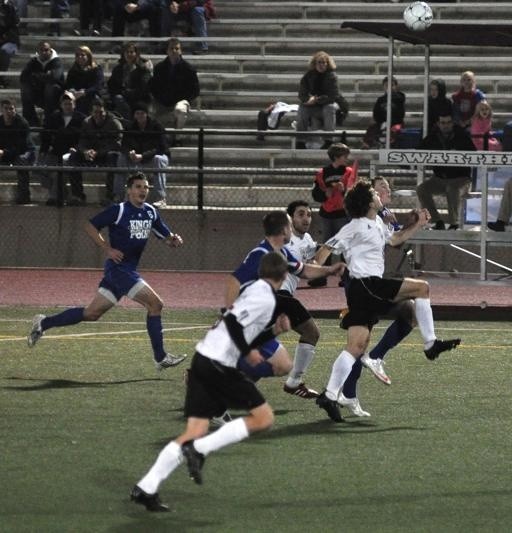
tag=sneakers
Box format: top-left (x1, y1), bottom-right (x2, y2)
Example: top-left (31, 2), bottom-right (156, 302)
top-left (152, 352), bottom-right (188, 371)
top-left (181, 438), bottom-right (204, 485)
top-left (129, 484), bottom-right (170, 512)
top-left (424, 338), bottom-right (461, 360)
top-left (210, 410), bottom-right (233, 428)
top-left (359, 353), bottom-right (391, 385)
top-left (283, 382), bottom-right (319, 398)
top-left (315, 389), bottom-right (344, 424)
top-left (152, 197), bottom-right (167, 207)
top-left (28, 313), bottom-right (47, 348)
top-left (336, 391), bottom-right (371, 417)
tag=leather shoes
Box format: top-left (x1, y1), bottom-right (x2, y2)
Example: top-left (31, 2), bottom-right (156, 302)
top-left (431, 219), bottom-right (444, 229)
top-left (488, 220), bottom-right (504, 230)
top-left (16, 192), bottom-right (31, 203)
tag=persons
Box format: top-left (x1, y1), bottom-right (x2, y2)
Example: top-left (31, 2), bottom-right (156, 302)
top-left (266, 199), bottom-right (322, 400)
top-left (427, 71), bottom-right (502, 151)
top-left (339, 174), bottom-right (424, 419)
top-left (416, 109), bottom-right (479, 231)
top-left (26, 167), bottom-right (189, 370)
top-left (222, 207), bottom-right (348, 388)
top-left (127, 249), bottom-right (294, 514)
top-left (0, 0), bottom-right (211, 206)
top-left (295, 52), bottom-right (343, 150)
top-left (362, 75), bottom-right (405, 150)
top-left (306, 140), bottom-right (358, 289)
top-left (303, 182), bottom-right (464, 425)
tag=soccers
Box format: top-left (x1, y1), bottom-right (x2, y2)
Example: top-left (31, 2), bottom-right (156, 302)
top-left (405, 0), bottom-right (433, 32)
